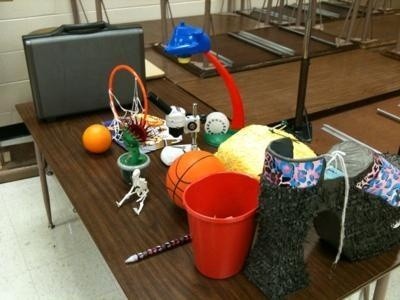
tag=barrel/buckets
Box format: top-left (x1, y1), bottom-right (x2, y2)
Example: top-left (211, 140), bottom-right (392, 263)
top-left (182, 172), bottom-right (262, 279)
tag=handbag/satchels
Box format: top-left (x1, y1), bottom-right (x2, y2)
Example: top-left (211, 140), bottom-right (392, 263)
top-left (22, 21), bottom-right (147, 120)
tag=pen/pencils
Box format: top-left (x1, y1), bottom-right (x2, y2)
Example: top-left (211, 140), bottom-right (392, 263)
top-left (125, 233), bottom-right (193, 264)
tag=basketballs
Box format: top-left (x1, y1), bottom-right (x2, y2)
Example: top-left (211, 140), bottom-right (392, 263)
top-left (83, 124), bottom-right (112, 154)
top-left (166, 151), bottom-right (226, 210)
